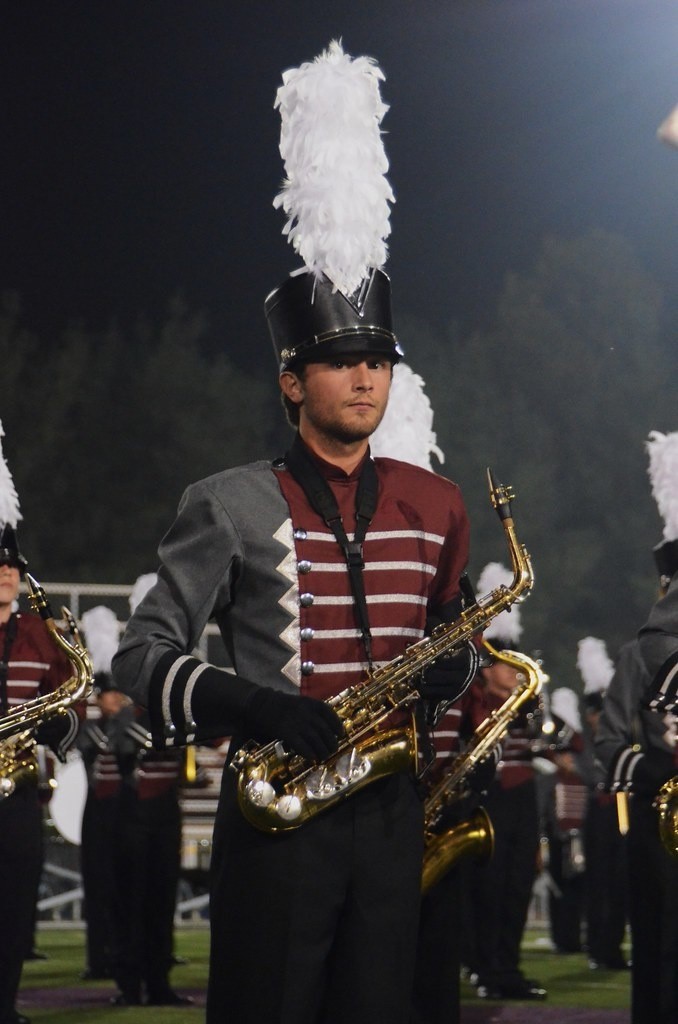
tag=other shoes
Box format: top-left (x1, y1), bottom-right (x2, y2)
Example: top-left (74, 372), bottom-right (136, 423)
top-left (80, 968), bottom-right (103, 980)
top-left (25, 948), bottom-right (47, 964)
top-left (146, 985), bottom-right (195, 1008)
top-left (544, 937), bottom-right (635, 974)
top-left (110, 986), bottom-right (143, 1006)
top-left (461, 966), bottom-right (549, 997)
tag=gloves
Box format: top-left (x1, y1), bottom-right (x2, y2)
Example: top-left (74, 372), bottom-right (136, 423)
top-left (150, 649), bottom-right (352, 768)
top-left (598, 747), bottom-right (674, 797)
top-left (507, 702), bottom-right (578, 751)
top-left (417, 614), bottom-right (478, 726)
top-left (34, 707), bottom-right (79, 764)
top-left (476, 747), bottom-right (503, 780)
top-left (124, 712), bottom-right (149, 749)
top-left (88, 703), bottom-right (131, 756)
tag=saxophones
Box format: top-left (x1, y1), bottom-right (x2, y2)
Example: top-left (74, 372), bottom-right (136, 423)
top-left (417, 570), bottom-right (543, 896)
top-left (231, 460), bottom-right (536, 839)
top-left (651, 774), bottom-right (678, 861)
top-left (1, 572), bottom-right (98, 804)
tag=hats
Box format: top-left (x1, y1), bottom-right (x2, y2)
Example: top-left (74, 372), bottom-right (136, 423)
top-left (0, 423), bottom-right (29, 575)
top-left (265, 37), bottom-right (407, 374)
top-left (470, 563), bottom-right (525, 665)
top-left (82, 605), bottom-right (125, 696)
top-left (643, 429), bottom-right (678, 596)
top-left (577, 638), bottom-right (617, 714)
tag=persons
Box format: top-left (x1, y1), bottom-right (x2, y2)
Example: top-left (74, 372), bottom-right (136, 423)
top-left (78, 362), bottom-right (675, 1024)
top-left (112, 33), bottom-right (484, 1024)
top-left (27, 926), bottom-right (49, 960)
top-left (0, 418), bottom-right (91, 1024)
